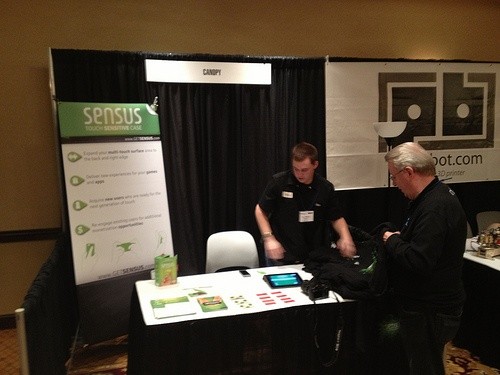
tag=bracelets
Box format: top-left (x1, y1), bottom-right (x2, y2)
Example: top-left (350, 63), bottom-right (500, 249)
top-left (262, 233), bottom-right (274, 239)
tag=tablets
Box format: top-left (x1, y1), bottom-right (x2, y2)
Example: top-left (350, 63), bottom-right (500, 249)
top-left (264, 272), bottom-right (303, 289)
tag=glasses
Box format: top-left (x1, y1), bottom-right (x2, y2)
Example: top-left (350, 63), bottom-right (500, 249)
top-left (389, 169), bottom-right (403, 182)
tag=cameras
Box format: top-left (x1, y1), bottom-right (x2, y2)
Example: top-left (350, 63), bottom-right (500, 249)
top-left (301, 279), bottom-right (329, 301)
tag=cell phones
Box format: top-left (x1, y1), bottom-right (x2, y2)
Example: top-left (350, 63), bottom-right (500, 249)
top-left (239, 270), bottom-right (251, 277)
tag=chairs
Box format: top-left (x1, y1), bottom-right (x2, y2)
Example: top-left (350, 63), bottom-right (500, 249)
top-left (467, 211), bottom-right (500, 238)
top-left (205, 231), bottom-right (259, 274)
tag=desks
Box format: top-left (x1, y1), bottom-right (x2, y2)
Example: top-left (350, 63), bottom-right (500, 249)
top-left (126, 264), bottom-right (409, 375)
top-left (450, 233), bottom-right (500, 370)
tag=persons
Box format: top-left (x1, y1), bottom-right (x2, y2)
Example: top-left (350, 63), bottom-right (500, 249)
top-left (380, 142), bottom-right (467, 375)
top-left (254, 143), bottom-right (356, 368)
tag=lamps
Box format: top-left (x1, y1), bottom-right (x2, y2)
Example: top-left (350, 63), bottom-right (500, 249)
top-left (372, 122), bottom-right (407, 186)
